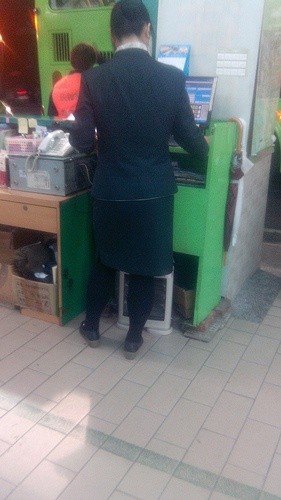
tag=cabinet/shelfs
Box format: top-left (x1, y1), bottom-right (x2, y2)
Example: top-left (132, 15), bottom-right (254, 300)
top-left (0, 190), bottom-right (89, 329)
top-left (168, 123), bottom-right (237, 326)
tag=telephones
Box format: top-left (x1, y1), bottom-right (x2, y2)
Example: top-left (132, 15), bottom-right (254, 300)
top-left (37, 129), bottom-right (73, 158)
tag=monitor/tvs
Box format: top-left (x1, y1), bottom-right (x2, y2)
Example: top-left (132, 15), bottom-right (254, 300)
top-left (186, 76), bottom-right (216, 127)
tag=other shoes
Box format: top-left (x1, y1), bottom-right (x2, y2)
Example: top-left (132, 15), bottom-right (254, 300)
top-left (80, 321), bottom-right (99, 348)
top-left (124, 336), bottom-right (144, 360)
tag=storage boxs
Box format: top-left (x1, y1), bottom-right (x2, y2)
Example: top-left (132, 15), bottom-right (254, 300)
top-left (6, 134), bottom-right (41, 154)
top-left (8, 153), bottom-right (91, 196)
top-left (173, 283), bottom-right (194, 319)
top-left (11, 274), bottom-right (59, 317)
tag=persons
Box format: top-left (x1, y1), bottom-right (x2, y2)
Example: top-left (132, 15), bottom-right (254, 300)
top-left (47, 43), bottom-right (97, 118)
top-left (69, 0), bottom-right (210, 359)
